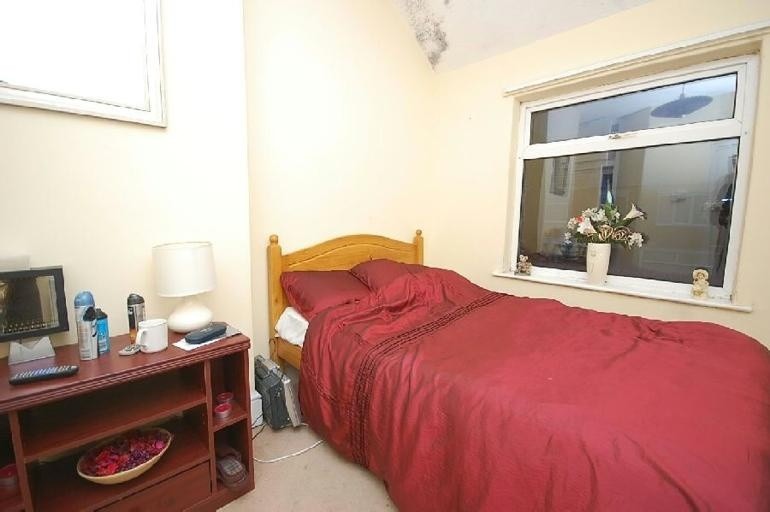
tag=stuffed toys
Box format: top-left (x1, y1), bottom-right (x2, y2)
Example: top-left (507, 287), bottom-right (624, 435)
top-left (515, 254), bottom-right (532, 275)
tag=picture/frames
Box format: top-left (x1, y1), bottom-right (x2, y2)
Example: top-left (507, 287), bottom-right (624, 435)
top-left (2, 1), bottom-right (168, 129)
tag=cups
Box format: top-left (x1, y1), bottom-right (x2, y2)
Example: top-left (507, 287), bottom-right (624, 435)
top-left (136, 318), bottom-right (169, 354)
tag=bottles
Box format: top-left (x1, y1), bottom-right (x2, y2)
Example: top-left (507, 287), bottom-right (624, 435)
top-left (127, 293), bottom-right (146, 344)
top-left (96, 308), bottom-right (111, 354)
top-left (75, 290), bottom-right (100, 361)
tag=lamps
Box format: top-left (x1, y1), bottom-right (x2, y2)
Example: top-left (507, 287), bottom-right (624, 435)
top-left (152, 239), bottom-right (219, 332)
top-left (651, 83), bottom-right (713, 121)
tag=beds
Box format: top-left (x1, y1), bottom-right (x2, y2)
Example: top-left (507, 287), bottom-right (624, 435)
top-left (262, 228), bottom-right (769, 512)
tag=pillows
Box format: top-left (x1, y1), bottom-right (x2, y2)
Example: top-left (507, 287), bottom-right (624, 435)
top-left (351, 259), bottom-right (434, 296)
top-left (281, 269), bottom-right (370, 321)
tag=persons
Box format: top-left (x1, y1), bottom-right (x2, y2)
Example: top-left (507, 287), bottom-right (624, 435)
top-left (690, 268), bottom-right (712, 298)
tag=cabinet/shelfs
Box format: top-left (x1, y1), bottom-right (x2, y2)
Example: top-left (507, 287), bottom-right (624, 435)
top-left (0, 321), bottom-right (257, 510)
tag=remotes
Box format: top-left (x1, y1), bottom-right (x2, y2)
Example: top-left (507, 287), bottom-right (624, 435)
top-left (119, 344), bottom-right (141, 355)
top-left (8, 365), bottom-right (78, 384)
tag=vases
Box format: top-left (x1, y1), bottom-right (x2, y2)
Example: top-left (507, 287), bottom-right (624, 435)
top-left (586, 242), bottom-right (611, 284)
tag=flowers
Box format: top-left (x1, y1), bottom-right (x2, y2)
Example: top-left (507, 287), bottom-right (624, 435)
top-left (561, 179), bottom-right (646, 250)
